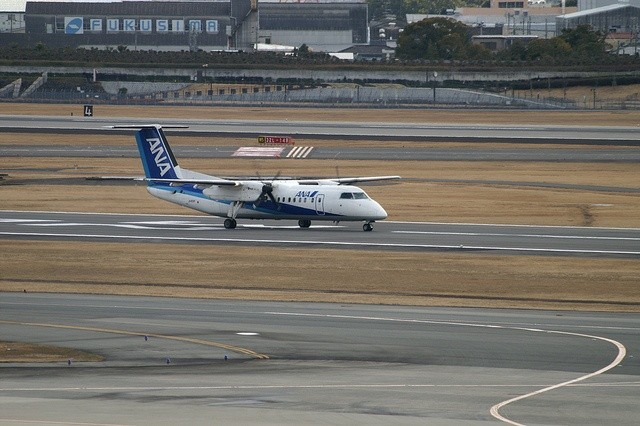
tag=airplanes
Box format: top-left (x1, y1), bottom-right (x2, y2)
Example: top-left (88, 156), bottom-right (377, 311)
top-left (83, 123), bottom-right (402, 236)
